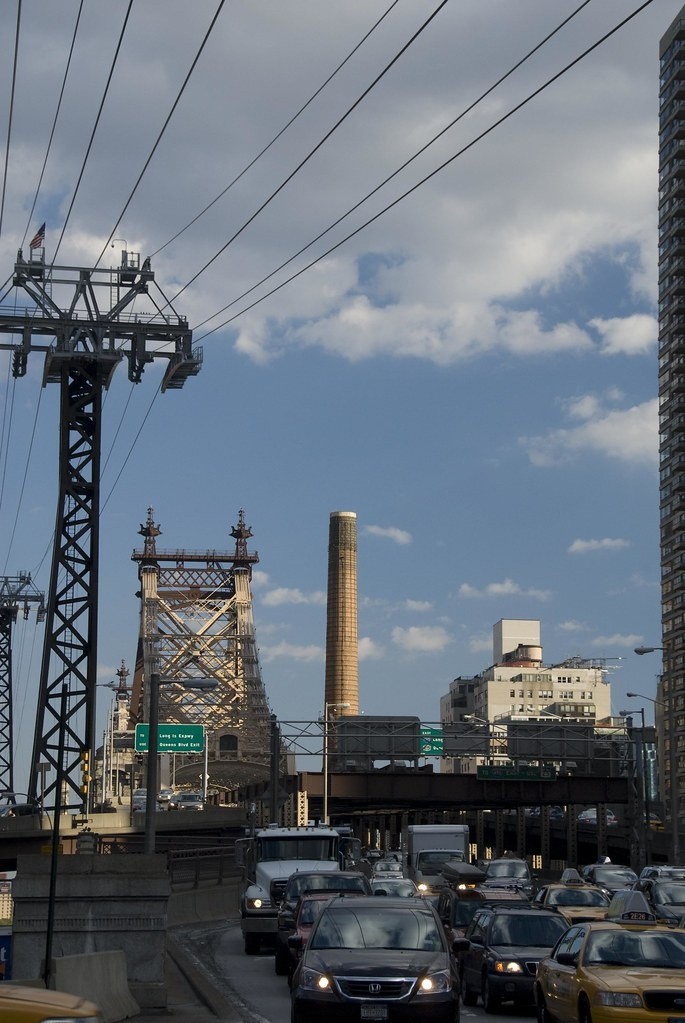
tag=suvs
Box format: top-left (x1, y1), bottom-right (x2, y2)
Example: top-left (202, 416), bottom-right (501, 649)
top-left (483, 850), bottom-right (535, 900)
top-left (640, 866), bottom-right (685, 888)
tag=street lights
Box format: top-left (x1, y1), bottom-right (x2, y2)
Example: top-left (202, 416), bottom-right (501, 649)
top-left (1, 792), bottom-right (58, 988)
top-left (143, 678), bottom-right (218, 853)
top-left (627, 646), bottom-right (680, 866)
top-left (619, 710), bottom-right (653, 866)
top-left (324, 703), bottom-right (348, 824)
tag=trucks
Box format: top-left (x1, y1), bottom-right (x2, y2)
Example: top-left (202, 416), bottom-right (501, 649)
top-left (401, 825), bottom-right (470, 894)
top-left (234, 823), bottom-right (362, 955)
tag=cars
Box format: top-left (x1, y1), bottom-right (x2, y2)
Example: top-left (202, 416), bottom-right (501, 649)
top-left (632, 876), bottom-right (685, 929)
top-left (582, 856), bottom-right (638, 898)
top-left (533, 890), bottom-right (685, 1023)
top-left (531, 868), bottom-right (611, 925)
top-left (273, 850), bottom-right (427, 974)
top-left (286, 893), bottom-right (473, 1023)
top-left (132, 788), bottom-right (204, 814)
top-left (0, 803), bottom-right (39, 817)
top-left (437, 886), bottom-right (531, 953)
